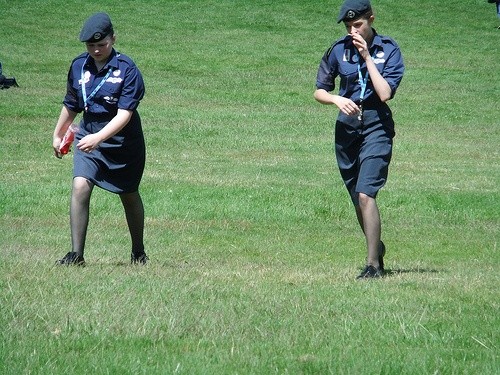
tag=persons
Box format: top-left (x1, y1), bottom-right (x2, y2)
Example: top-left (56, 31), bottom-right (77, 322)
top-left (51, 11), bottom-right (150, 267)
top-left (312, 0), bottom-right (406, 281)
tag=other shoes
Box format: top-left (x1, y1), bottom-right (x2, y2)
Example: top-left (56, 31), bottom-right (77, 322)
top-left (130, 252), bottom-right (148, 266)
top-left (60, 251), bottom-right (85, 268)
top-left (378, 240), bottom-right (385, 272)
top-left (356, 263), bottom-right (384, 280)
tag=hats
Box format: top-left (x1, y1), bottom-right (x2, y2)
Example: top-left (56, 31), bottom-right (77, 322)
top-left (80, 13), bottom-right (112, 43)
top-left (337, 0), bottom-right (371, 23)
